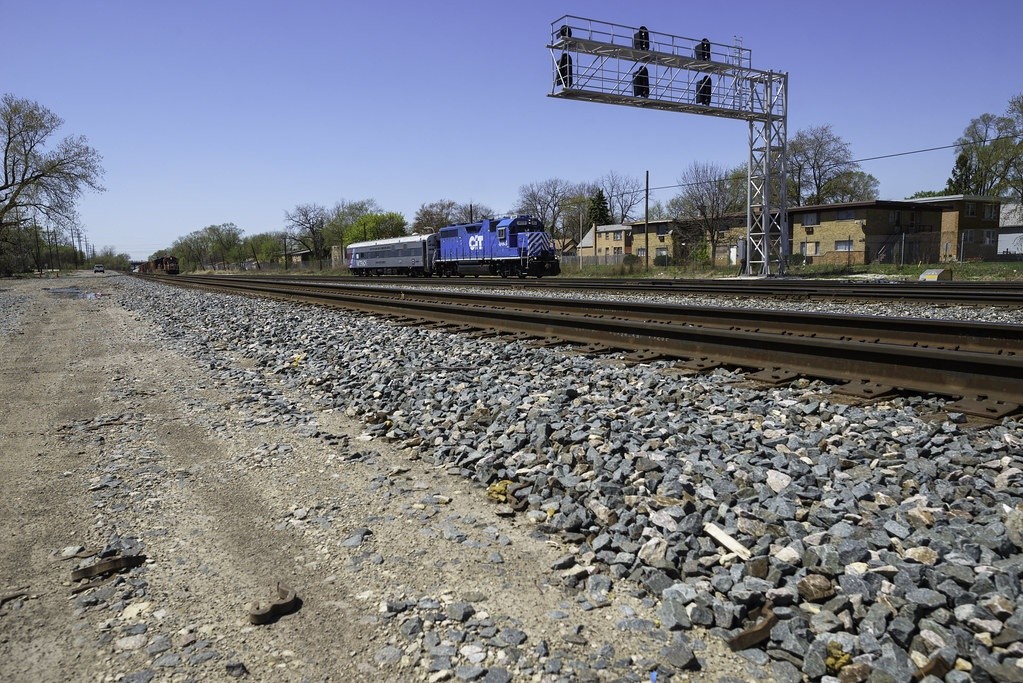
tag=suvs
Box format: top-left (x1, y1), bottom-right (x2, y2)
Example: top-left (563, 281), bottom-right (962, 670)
top-left (94, 264), bottom-right (104, 273)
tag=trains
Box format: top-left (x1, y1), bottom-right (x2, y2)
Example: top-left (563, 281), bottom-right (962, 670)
top-left (346, 214), bottom-right (561, 279)
top-left (133, 256), bottom-right (180, 275)
